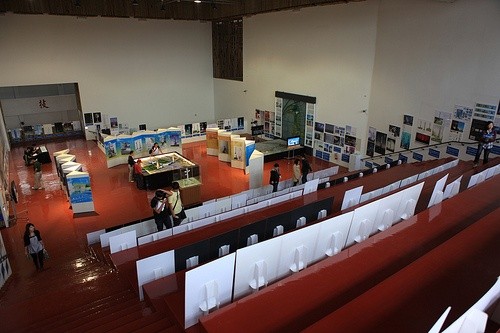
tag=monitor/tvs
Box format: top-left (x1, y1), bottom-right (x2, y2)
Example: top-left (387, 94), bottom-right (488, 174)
top-left (287, 136), bottom-right (300, 146)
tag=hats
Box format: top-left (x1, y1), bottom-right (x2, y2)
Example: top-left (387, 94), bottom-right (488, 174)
top-left (137, 159), bottom-right (142, 163)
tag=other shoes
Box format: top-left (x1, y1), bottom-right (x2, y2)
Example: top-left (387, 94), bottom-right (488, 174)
top-left (32, 187), bottom-right (35, 189)
top-left (129, 180), bottom-right (134, 182)
top-left (39, 187), bottom-right (42, 189)
top-left (36, 265), bottom-right (39, 270)
top-left (473, 163), bottom-right (477, 168)
top-left (40, 265), bottom-right (43, 271)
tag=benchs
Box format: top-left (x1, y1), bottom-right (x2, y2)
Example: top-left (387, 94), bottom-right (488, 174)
top-left (86, 156), bottom-right (500, 333)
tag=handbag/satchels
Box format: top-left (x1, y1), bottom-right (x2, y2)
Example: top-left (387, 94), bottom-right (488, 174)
top-left (43, 249), bottom-right (49, 260)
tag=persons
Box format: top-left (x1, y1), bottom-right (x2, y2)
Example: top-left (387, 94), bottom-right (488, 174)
top-left (149, 150), bottom-right (154, 156)
top-left (151, 189), bottom-right (173, 232)
top-left (473, 121), bottom-right (497, 167)
top-left (24, 223), bottom-right (47, 272)
top-left (269, 163), bottom-right (281, 193)
top-left (127, 151), bottom-right (137, 183)
top-left (293, 159), bottom-right (301, 186)
top-left (19, 121), bottom-right (28, 142)
top-left (152, 143), bottom-right (163, 155)
top-left (167, 182), bottom-right (187, 226)
top-left (300, 153), bottom-right (312, 184)
top-left (28, 158), bottom-right (42, 189)
